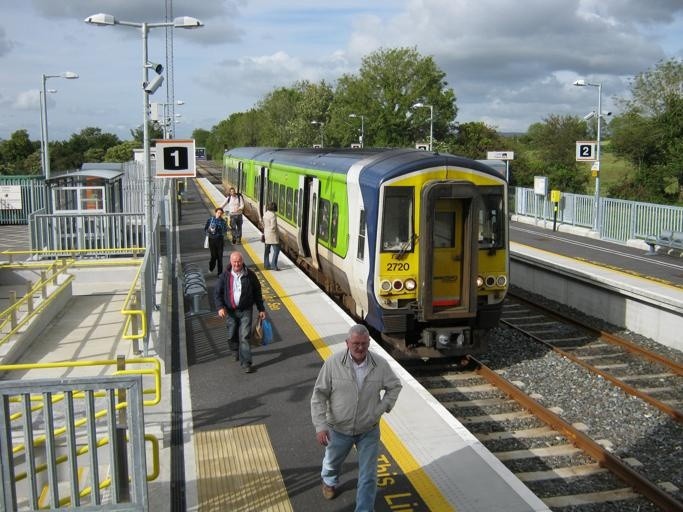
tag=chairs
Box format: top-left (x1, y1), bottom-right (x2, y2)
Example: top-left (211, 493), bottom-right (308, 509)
top-left (646, 227), bottom-right (682, 261)
top-left (174, 258), bottom-right (210, 318)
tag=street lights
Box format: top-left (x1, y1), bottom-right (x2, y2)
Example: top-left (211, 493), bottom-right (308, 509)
top-left (84, 13), bottom-right (205, 243)
top-left (40, 71), bottom-right (80, 180)
top-left (413, 102), bottom-right (433, 152)
top-left (348, 114), bottom-right (364, 149)
top-left (572, 79), bottom-right (612, 197)
top-left (311, 121), bottom-right (324, 149)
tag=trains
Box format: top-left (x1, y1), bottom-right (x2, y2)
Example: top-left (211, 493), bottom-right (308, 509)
top-left (222, 145), bottom-right (511, 363)
top-left (195, 147), bottom-right (207, 160)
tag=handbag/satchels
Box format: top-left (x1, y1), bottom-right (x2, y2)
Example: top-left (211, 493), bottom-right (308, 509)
top-left (203, 235), bottom-right (210, 250)
top-left (254, 318), bottom-right (273, 348)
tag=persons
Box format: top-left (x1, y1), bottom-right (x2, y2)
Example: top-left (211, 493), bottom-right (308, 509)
top-left (263, 202), bottom-right (280, 271)
top-left (220, 187), bottom-right (244, 245)
top-left (213, 251), bottom-right (266, 373)
top-left (204, 208), bottom-right (227, 280)
top-left (310, 324), bottom-right (402, 512)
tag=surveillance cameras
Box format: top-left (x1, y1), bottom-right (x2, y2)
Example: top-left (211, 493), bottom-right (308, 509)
top-left (146, 55), bottom-right (165, 76)
top-left (144, 73), bottom-right (165, 97)
top-left (601, 109), bottom-right (612, 117)
top-left (583, 110), bottom-right (594, 122)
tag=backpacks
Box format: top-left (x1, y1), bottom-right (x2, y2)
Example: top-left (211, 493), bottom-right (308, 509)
top-left (228, 192), bottom-right (243, 203)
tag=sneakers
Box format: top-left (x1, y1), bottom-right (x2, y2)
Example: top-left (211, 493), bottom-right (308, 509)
top-left (230, 350), bottom-right (238, 362)
top-left (265, 264), bottom-right (282, 272)
top-left (320, 478), bottom-right (335, 500)
top-left (242, 361), bottom-right (252, 373)
